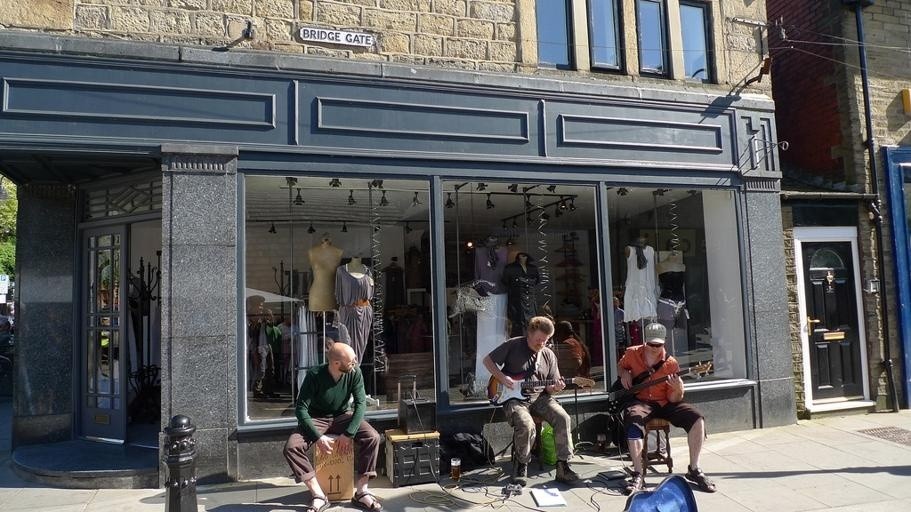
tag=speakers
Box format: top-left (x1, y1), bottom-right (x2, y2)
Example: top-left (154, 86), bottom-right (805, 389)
top-left (400, 397), bottom-right (437, 435)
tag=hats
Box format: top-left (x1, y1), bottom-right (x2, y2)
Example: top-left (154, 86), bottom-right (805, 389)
top-left (644, 322), bottom-right (669, 345)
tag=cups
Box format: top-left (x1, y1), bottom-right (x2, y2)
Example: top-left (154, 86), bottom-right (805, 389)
top-left (451, 457), bottom-right (461, 483)
top-left (598, 433), bottom-right (606, 448)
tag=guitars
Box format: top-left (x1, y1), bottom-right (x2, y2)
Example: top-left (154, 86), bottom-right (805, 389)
top-left (608, 361), bottom-right (712, 414)
top-left (488, 372), bottom-right (596, 406)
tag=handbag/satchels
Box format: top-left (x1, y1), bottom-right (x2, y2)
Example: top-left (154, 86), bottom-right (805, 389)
top-left (439, 429), bottom-right (497, 473)
top-left (537, 416), bottom-right (561, 467)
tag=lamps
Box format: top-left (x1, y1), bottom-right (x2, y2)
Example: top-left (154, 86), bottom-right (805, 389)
top-left (615, 186), bottom-right (662, 199)
top-left (267, 176), bottom-right (424, 236)
top-left (442, 178), bottom-right (578, 247)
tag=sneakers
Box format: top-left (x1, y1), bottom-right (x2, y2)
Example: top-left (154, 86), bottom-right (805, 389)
top-left (264, 391), bottom-right (281, 398)
top-left (254, 391), bottom-right (271, 399)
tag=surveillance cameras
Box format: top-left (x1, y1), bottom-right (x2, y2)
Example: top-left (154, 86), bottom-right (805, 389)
top-left (761, 57), bottom-right (775, 75)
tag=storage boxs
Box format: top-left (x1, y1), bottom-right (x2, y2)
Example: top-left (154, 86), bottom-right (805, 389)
top-left (311, 433), bottom-right (354, 502)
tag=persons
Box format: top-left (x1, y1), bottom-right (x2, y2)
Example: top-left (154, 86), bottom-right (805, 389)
top-left (246, 295), bottom-right (294, 400)
top-left (483, 316), bottom-right (580, 487)
top-left (307, 238), bottom-right (375, 367)
top-left (283, 343), bottom-right (383, 512)
top-left (615, 322), bottom-right (717, 495)
top-left (473, 236), bottom-right (508, 285)
top-left (622, 237), bottom-right (658, 322)
top-left (558, 287), bottom-right (624, 382)
top-left (490, 252), bottom-right (540, 338)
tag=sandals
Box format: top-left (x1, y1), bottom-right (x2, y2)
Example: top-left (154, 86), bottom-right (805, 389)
top-left (685, 463), bottom-right (720, 493)
top-left (625, 471), bottom-right (646, 496)
top-left (305, 495), bottom-right (332, 512)
top-left (351, 490), bottom-right (385, 512)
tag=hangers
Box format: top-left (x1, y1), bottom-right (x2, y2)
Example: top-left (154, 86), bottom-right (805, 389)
top-left (551, 238), bottom-right (585, 298)
top-left (380, 257), bottom-right (405, 271)
top-left (385, 303), bottom-right (417, 319)
top-left (243, 314), bottom-right (292, 330)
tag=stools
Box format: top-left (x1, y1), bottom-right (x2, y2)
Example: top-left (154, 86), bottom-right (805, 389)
top-left (639, 418), bottom-right (673, 479)
top-left (508, 415), bottom-right (545, 472)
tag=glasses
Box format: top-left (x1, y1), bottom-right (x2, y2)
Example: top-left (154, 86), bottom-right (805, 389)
top-left (647, 341), bottom-right (665, 348)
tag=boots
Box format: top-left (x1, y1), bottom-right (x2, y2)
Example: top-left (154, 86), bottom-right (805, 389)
top-left (555, 460), bottom-right (581, 485)
top-left (512, 455), bottom-right (530, 486)
